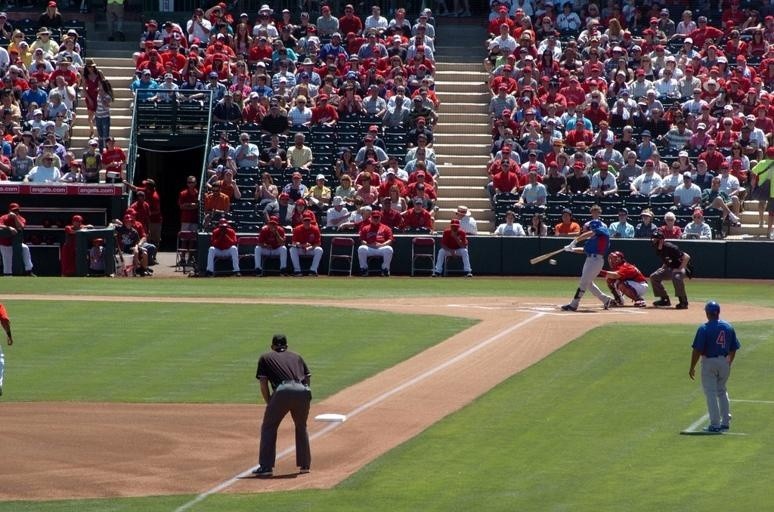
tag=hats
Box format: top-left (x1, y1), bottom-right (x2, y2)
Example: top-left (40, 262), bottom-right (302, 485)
top-left (142, 178), bottom-right (156, 189)
top-left (72, 215), bottom-right (83, 222)
top-left (9, 203), bottom-right (20, 211)
top-left (449, 219), bottom-right (460, 227)
top-left (452, 205), bottom-right (472, 217)
top-left (216, 115), bottom-right (426, 228)
top-left (143, 2), bottom-right (429, 105)
top-left (488, 0), bottom-right (774, 221)
top-left (123, 207), bottom-right (136, 221)
top-left (0, 1), bottom-right (116, 168)
top-left (273, 335), bottom-right (287, 344)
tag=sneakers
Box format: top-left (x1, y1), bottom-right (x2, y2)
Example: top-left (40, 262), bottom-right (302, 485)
top-left (653, 297), bottom-right (672, 309)
top-left (676, 301), bottom-right (689, 309)
top-left (280, 269), bottom-right (288, 277)
top-left (294, 271), bottom-right (303, 277)
top-left (561, 304), bottom-right (578, 312)
top-left (25, 270), bottom-right (37, 277)
top-left (252, 465), bottom-right (274, 477)
top-left (188, 259), bottom-right (197, 266)
top-left (176, 259), bottom-right (186, 266)
top-left (610, 297), bottom-right (625, 307)
top-left (604, 297), bottom-right (611, 309)
top-left (235, 271), bottom-right (241, 277)
top-left (381, 270), bottom-right (391, 277)
top-left (701, 424), bottom-right (730, 434)
top-left (3, 272), bottom-right (13, 277)
top-left (464, 272), bottom-right (473, 277)
top-left (308, 271), bottom-right (318, 277)
top-left (115, 31), bottom-right (125, 42)
top-left (153, 258), bottom-right (160, 264)
top-left (360, 268), bottom-right (369, 277)
top-left (432, 271), bottom-right (441, 277)
top-left (633, 300), bottom-right (646, 307)
top-left (140, 267), bottom-right (154, 277)
top-left (300, 466), bottom-right (310, 473)
top-left (204, 270), bottom-right (214, 277)
top-left (256, 269), bottom-right (263, 277)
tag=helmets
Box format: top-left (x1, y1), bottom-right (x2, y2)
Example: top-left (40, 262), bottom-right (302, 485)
top-left (651, 231), bottom-right (666, 247)
top-left (46, 234), bottom-right (55, 245)
top-left (43, 220), bottom-right (50, 228)
top-left (588, 220), bottom-right (603, 232)
top-left (56, 220), bottom-right (65, 228)
top-left (704, 301), bottom-right (720, 313)
top-left (92, 238), bottom-right (104, 247)
top-left (30, 234), bottom-right (42, 245)
top-left (608, 251), bottom-right (624, 269)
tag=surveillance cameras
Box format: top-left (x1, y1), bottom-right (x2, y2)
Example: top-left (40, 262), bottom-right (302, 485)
top-left (107, 171), bottom-right (121, 178)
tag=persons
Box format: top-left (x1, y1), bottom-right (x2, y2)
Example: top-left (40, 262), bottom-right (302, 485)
top-left (551, 218), bottom-right (617, 311)
top-left (483, 1), bottom-right (774, 241)
top-left (597, 251), bottom-right (650, 310)
top-left (0, 304), bottom-right (13, 397)
top-left (249, 332), bottom-right (314, 476)
top-left (687, 301), bottom-right (743, 435)
top-left (0, 0), bottom-right (442, 275)
top-left (432, 205), bottom-right (477, 274)
top-left (648, 228), bottom-right (693, 310)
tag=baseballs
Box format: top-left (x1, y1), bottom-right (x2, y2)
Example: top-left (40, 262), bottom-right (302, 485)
top-left (550, 260), bottom-right (556, 265)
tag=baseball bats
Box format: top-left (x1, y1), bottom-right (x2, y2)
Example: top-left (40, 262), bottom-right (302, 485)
top-left (530, 246), bottom-right (566, 264)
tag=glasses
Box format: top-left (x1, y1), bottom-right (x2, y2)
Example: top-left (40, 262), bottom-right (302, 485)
top-left (212, 190), bottom-right (218, 192)
